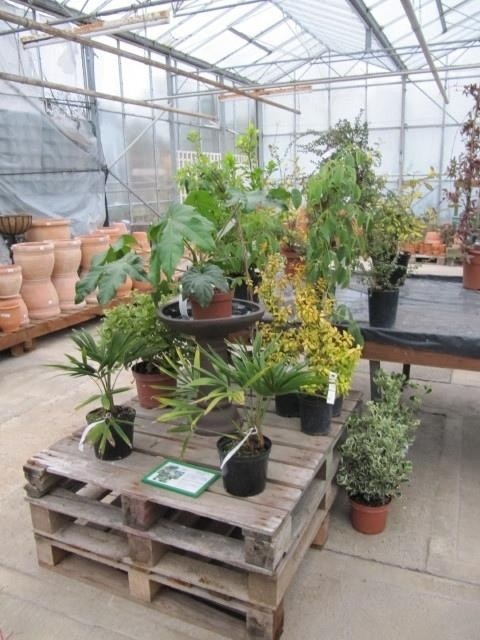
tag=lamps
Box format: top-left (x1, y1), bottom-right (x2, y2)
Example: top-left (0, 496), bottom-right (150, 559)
top-left (21, 9), bottom-right (171, 51)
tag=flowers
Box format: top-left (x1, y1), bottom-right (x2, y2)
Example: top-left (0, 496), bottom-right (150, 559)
top-left (435, 81), bottom-right (479, 254)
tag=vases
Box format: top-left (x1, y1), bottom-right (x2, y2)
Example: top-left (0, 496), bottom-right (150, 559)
top-left (458, 247), bottom-right (480, 291)
top-left (0, 214), bottom-right (166, 334)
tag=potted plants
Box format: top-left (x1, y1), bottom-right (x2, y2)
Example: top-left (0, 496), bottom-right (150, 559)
top-left (40, 327), bottom-right (171, 459)
top-left (336, 370), bottom-right (431, 534)
top-left (153, 107), bottom-right (444, 497)
top-left (74, 206), bottom-right (240, 410)
